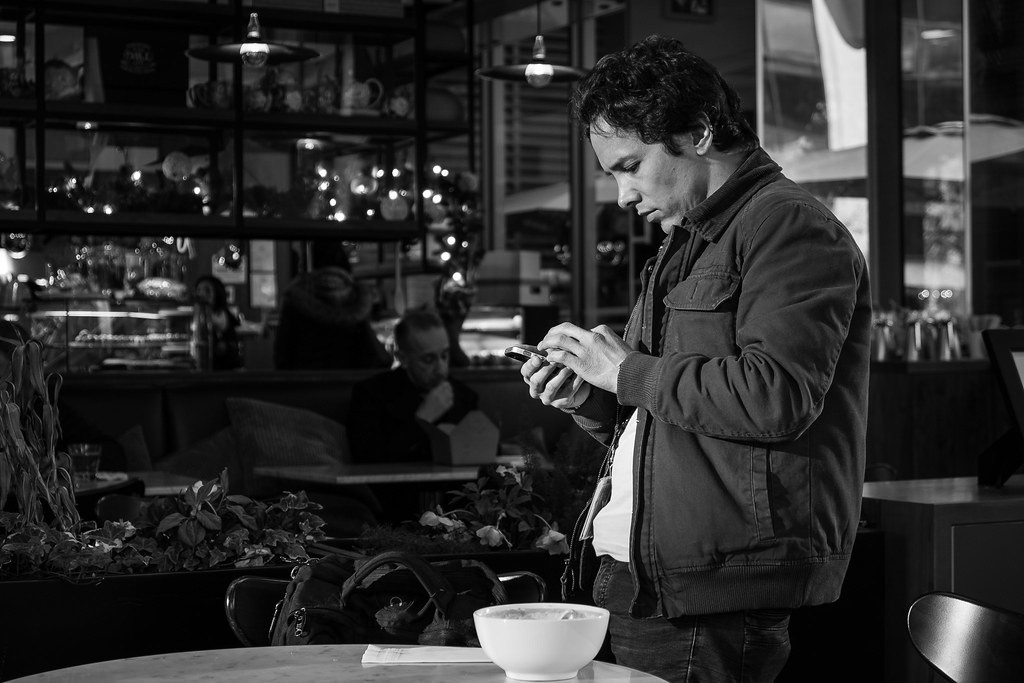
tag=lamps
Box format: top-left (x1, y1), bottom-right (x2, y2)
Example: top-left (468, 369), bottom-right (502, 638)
top-left (474, 0), bottom-right (589, 88)
top-left (184, 0), bottom-right (319, 66)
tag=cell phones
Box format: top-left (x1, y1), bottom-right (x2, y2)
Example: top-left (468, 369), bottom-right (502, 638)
top-left (505, 346), bottom-right (566, 374)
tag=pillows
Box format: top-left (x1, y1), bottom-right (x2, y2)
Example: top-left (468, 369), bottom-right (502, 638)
top-left (217, 396), bottom-right (349, 498)
top-left (169, 429), bottom-right (241, 485)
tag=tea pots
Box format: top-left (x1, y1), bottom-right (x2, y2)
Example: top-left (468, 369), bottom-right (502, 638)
top-left (190, 72), bottom-right (383, 117)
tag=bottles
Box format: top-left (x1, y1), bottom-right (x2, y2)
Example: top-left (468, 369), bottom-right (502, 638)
top-left (870, 312), bottom-right (997, 362)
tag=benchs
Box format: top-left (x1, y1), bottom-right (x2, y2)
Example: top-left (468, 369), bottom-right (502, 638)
top-left (48, 375), bottom-right (619, 468)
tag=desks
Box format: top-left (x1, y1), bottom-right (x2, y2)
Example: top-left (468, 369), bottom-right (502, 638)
top-left (254, 466), bottom-right (491, 483)
top-left (5, 644), bottom-right (673, 682)
top-left (861, 472), bottom-right (1024, 683)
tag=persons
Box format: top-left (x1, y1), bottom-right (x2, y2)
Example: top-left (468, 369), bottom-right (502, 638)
top-left (521, 33), bottom-right (874, 683)
top-left (346, 314), bottom-right (481, 526)
top-left (197, 275), bottom-right (240, 372)
top-left (276, 269), bottom-right (393, 421)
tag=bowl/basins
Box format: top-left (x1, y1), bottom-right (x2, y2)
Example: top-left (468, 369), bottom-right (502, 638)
top-left (472, 603), bottom-right (610, 681)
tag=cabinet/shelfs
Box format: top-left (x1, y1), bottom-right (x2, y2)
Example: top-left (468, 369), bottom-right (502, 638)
top-left (0, 0), bottom-right (432, 244)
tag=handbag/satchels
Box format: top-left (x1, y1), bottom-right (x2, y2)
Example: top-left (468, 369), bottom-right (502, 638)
top-left (272, 551), bottom-right (511, 646)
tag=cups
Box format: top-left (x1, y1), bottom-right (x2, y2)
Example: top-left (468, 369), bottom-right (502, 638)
top-left (69, 444), bottom-right (103, 480)
